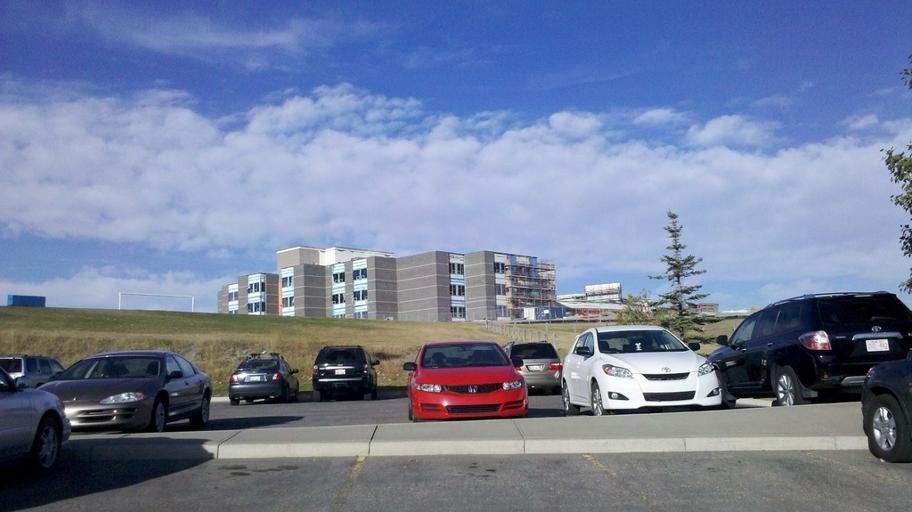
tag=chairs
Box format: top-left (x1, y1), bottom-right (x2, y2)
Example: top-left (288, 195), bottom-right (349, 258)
top-left (147, 362), bottom-right (159, 376)
top-left (473, 350), bottom-right (498, 366)
top-left (430, 352), bottom-right (452, 367)
top-left (101, 364), bottom-right (128, 378)
top-left (599, 340), bottom-right (622, 353)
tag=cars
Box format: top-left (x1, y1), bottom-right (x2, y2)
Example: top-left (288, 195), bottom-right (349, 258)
top-left (557, 323), bottom-right (728, 417)
top-left (401, 338), bottom-right (530, 423)
top-left (33, 348), bottom-right (215, 441)
top-left (859, 343), bottom-right (912, 466)
top-left (1, 364), bottom-right (72, 496)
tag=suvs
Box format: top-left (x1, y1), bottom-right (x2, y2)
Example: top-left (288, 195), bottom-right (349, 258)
top-left (308, 344), bottom-right (382, 401)
top-left (0, 353), bottom-right (66, 396)
top-left (505, 338), bottom-right (564, 398)
top-left (226, 347), bottom-right (302, 406)
top-left (702, 288), bottom-right (912, 405)
